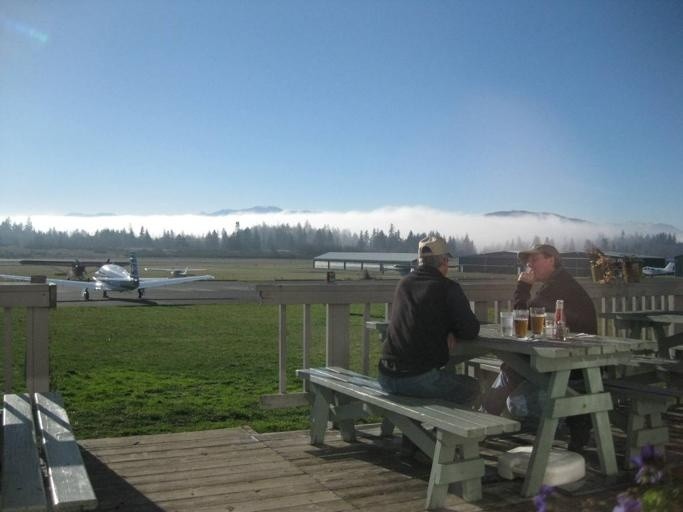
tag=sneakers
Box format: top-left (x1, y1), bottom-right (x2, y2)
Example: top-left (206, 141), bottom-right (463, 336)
top-left (565, 417), bottom-right (595, 453)
top-left (393, 457), bottom-right (414, 476)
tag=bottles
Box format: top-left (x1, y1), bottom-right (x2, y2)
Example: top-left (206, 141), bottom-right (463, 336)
top-left (554, 300), bottom-right (566, 340)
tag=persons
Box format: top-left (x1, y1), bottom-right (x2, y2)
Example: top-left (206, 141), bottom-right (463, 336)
top-left (377, 237), bottom-right (481, 478)
top-left (478, 244), bottom-right (598, 453)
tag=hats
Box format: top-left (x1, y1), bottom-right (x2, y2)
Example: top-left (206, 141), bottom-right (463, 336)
top-left (416, 236), bottom-right (454, 262)
top-left (515, 243), bottom-right (561, 262)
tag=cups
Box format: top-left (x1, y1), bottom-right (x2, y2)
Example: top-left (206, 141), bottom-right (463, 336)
top-left (499, 309), bottom-right (529, 339)
top-left (545, 312), bottom-right (553, 337)
top-left (530, 307), bottom-right (545, 338)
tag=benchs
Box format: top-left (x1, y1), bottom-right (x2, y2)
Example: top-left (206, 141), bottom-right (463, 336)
top-left (464, 355), bottom-right (679, 470)
top-left (1, 386), bottom-right (100, 511)
top-left (293, 363), bottom-right (523, 512)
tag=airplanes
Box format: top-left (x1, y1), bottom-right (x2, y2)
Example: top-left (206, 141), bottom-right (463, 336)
top-left (642, 260), bottom-right (677, 277)
top-left (1, 248), bottom-right (216, 304)
